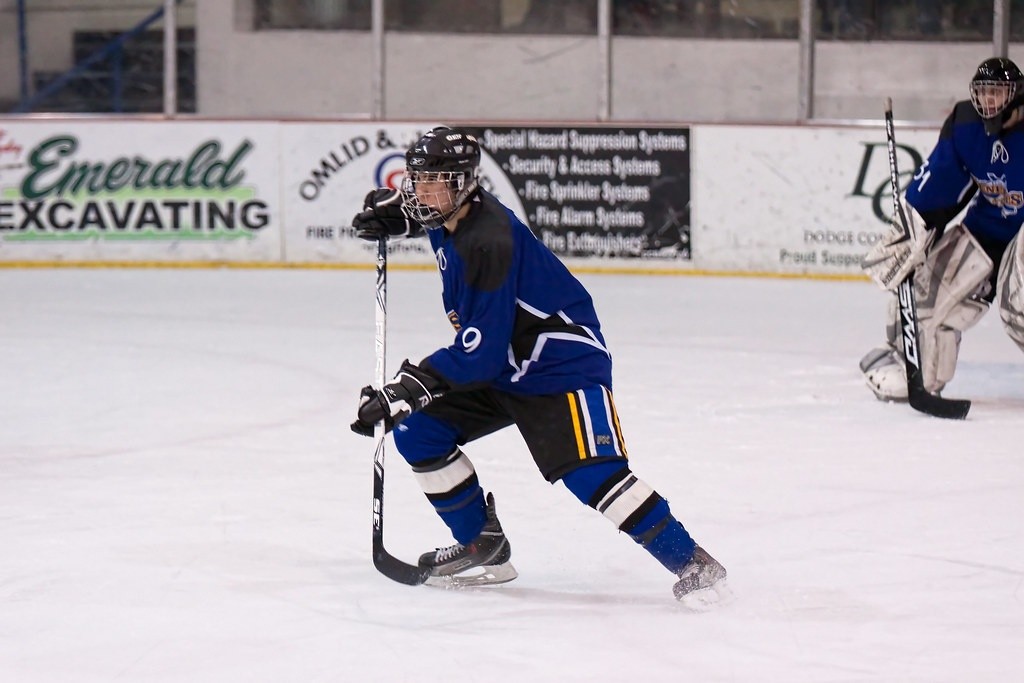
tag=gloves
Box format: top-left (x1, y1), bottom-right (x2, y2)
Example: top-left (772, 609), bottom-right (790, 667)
top-left (351, 358), bottom-right (446, 438)
top-left (351, 186), bottom-right (424, 238)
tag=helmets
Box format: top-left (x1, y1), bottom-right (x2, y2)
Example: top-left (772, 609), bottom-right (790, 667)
top-left (968, 57), bottom-right (1024, 125)
top-left (402, 126), bottom-right (481, 231)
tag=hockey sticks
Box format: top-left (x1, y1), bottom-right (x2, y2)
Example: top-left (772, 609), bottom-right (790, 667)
top-left (371, 237), bottom-right (437, 585)
top-left (884, 96), bottom-right (973, 422)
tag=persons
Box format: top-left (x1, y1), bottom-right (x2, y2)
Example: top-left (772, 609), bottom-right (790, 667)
top-left (861, 58), bottom-right (1024, 403)
top-left (352, 127), bottom-right (732, 610)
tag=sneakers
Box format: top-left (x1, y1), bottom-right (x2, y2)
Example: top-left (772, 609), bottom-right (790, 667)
top-left (672, 545), bottom-right (729, 611)
top-left (418, 491), bottom-right (518, 585)
